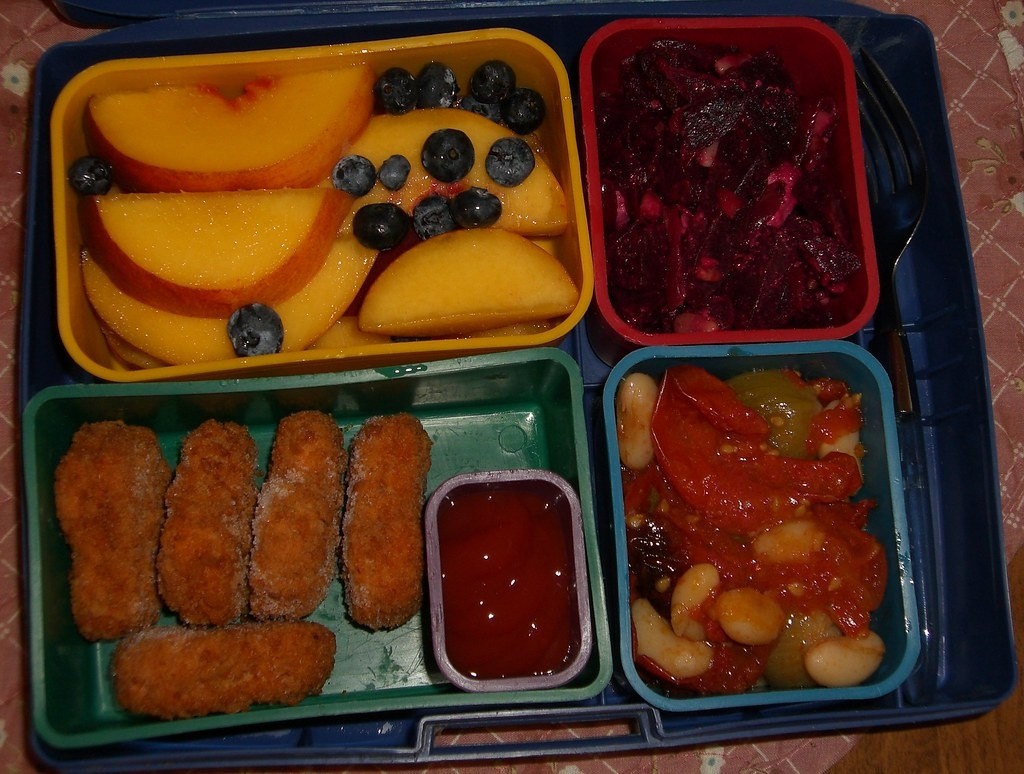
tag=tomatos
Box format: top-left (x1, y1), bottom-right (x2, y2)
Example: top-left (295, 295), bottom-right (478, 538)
top-left (629, 363), bottom-right (887, 693)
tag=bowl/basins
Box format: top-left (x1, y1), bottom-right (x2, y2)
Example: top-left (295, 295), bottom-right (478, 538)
top-left (428, 462), bottom-right (595, 693)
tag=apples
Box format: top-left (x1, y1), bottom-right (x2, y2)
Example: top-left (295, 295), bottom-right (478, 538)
top-left (81, 60), bottom-right (580, 367)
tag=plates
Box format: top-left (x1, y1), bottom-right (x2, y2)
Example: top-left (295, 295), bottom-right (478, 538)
top-left (50, 29), bottom-right (593, 384)
top-left (578, 21), bottom-right (882, 347)
top-left (603, 340), bottom-right (919, 713)
top-left (28, 344), bottom-right (620, 751)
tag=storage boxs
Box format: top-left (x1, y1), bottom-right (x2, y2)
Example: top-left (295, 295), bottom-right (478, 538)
top-left (54, 28), bottom-right (596, 387)
top-left (600, 339), bottom-right (922, 714)
top-left (23, 348), bottom-right (614, 750)
top-left (578, 16), bottom-right (877, 373)
